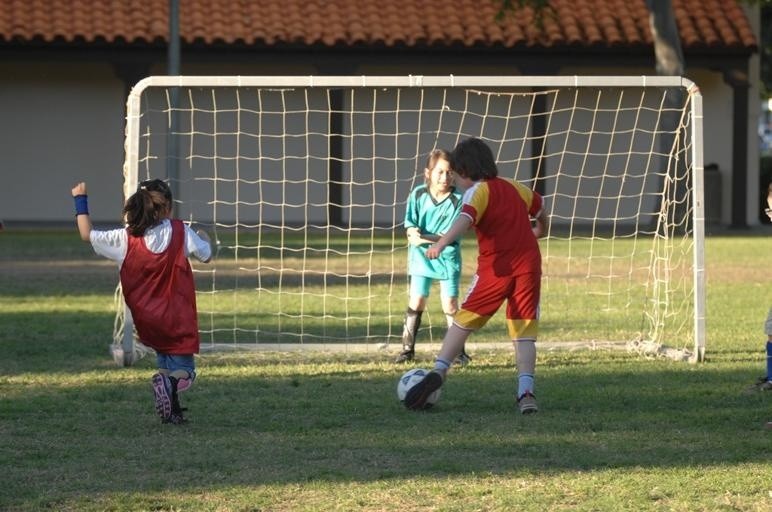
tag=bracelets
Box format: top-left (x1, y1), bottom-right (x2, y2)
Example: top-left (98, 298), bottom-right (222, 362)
top-left (73, 193), bottom-right (90, 217)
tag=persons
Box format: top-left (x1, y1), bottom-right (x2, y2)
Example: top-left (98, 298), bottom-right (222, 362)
top-left (395, 149), bottom-right (472, 364)
top-left (405, 137), bottom-right (546, 414)
top-left (70, 182), bottom-right (213, 425)
top-left (749, 190), bottom-right (772, 395)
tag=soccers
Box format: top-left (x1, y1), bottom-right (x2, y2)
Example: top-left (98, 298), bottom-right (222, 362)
top-left (398, 369), bottom-right (441, 409)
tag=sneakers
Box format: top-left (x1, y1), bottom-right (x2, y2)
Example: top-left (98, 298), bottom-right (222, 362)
top-left (400, 366), bottom-right (448, 410)
top-left (516, 389), bottom-right (540, 414)
top-left (450, 353), bottom-right (470, 368)
top-left (150, 371), bottom-right (184, 426)
top-left (394, 349), bottom-right (415, 365)
top-left (744, 379), bottom-right (772, 396)
